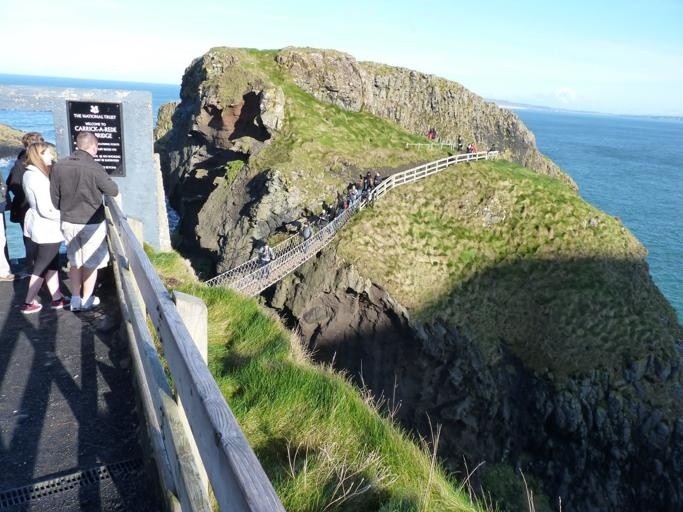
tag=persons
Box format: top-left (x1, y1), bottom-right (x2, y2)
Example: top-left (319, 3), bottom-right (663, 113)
top-left (303, 222), bottom-right (313, 255)
top-left (490, 144), bottom-right (496, 151)
top-left (0, 131), bottom-right (119, 314)
top-left (426, 128), bottom-right (436, 140)
top-left (316, 172), bottom-right (382, 241)
top-left (258, 242), bottom-right (275, 279)
top-left (457, 135), bottom-right (463, 151)
top-left (467, 142), bottom-right (477, 153)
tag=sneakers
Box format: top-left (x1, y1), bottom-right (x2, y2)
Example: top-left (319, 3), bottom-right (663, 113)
top-left (70, 295), bottom-right (101, 312)
top-left (51, 296), bottom-right (69, 309)
top-left (0, 274), bottom-right (25, 283)
top-left (19, 302), bottom-right (44, 315)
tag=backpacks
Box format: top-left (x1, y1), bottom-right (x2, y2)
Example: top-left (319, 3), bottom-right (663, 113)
top-left (6, 157), bottom-right (29, 187)
